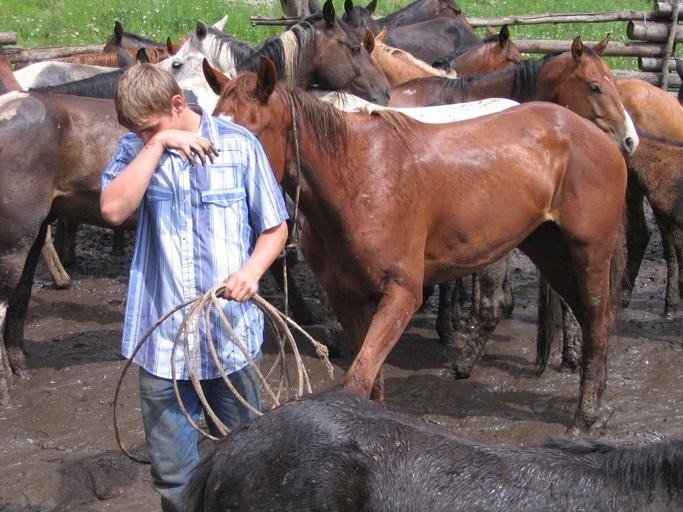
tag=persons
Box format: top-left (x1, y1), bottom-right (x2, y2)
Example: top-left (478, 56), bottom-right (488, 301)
top-left (99, 63), bottom-right (292, 512)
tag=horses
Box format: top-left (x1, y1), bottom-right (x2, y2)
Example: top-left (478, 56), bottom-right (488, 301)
top-left (179, 389), bottom-right (683, 512)
top-left (0, 0), bottom-right (683, 444)
